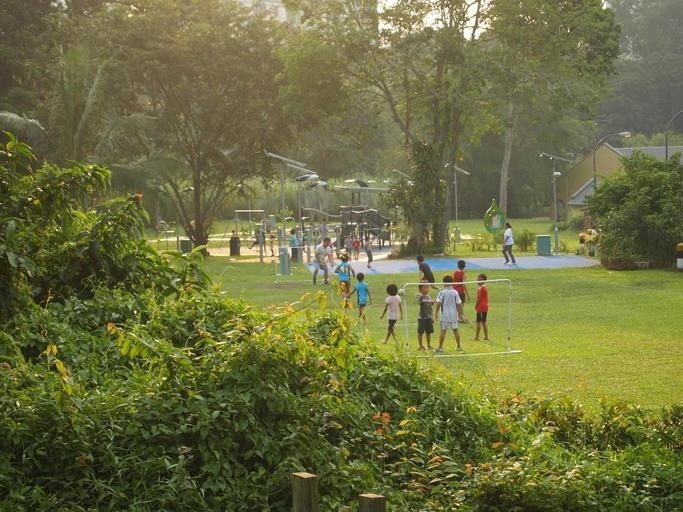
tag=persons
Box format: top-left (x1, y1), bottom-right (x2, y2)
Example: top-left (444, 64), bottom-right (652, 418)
top-left (452, 258), bottom-right (469, 323)
top-left (269, 234), bottom-right (277, 256)
top-left (418, 280), bottom-right (434, 351)
top-left (416, 255), bottom-right (441, 292)
top-left (472, 273), bottom-right (489, 342)
top-left (231, 229), bottom-right (236, 237)
top-left (288, 214), bottom-right (375, 323)
top-left (380, 283), bottom-right (403, 346)
top-left (248, 230), bottom-right (267, 254)
top-left (501, 221), bottom-right (518, 265)
top-left (433, 275), bottom-right (465, 352)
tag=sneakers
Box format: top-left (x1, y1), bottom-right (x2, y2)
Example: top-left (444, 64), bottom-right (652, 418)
top-left (455, 347), bottom-right (463, 352)
top-left (419, 346), bottom-right (426, 351)
top-left (434, 347), bottom-right (444, 353)
top-left (426, 345), bottom-right (433, 350)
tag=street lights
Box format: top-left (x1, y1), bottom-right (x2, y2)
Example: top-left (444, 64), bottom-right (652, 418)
top-left (444, 160), bottom-right (472, 225)
top-left (279, 173), bottom-right (318, 275)
top-left (392, 168), bottom-right (410, 182)
top-left (665, 111), bottom-right (682, 160)
top-left (299, 179), bottom-right (326, 250)
top-left (537, 151), bottom-right (573, 254)
top-left (592, 131), bottom-right (631, 196)
top-left (231, 182), bottom-right (242, 234)
top-left (175, 187), bottom-right (193, 251)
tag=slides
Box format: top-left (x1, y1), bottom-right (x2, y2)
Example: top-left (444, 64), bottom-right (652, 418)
top-left (332, 210), bottom-right (391, 251)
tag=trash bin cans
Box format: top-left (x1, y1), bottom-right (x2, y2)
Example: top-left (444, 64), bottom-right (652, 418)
top-left (279, 248), bottom-right (289, 274)
top-left (181, 240), bottom-right (192, 253)
top-left (229, 237), bottom-right (241, 257)
top-left (537, 235), bottom-right (551, 256)
top-left (292, 248), bottom-right (302, 264)
